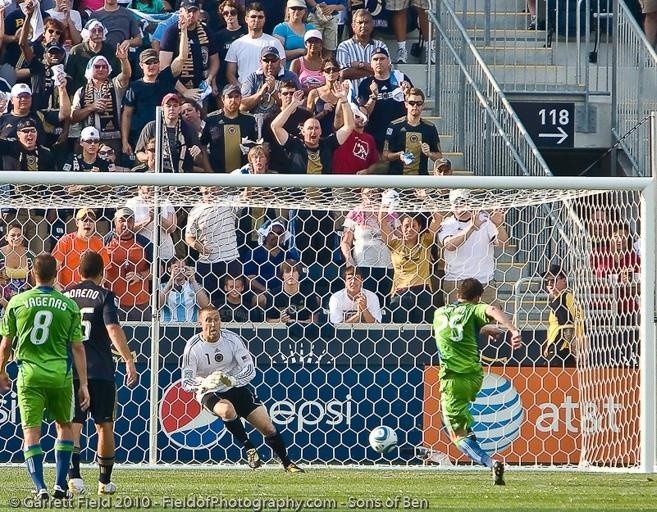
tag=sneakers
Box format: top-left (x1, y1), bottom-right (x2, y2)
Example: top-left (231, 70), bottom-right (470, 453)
top-left (34, 489), bottom-right (49, 501)
top-left (246, 447), bottom-right (260, 468)
top-left (68, 479), bottom-right (86, 495)
top-left (98, 480), bottom-right (116, 496)
top-left (493, 461), bottom-right (505, 485)
top-left (285, 463), bottom-right (304, 472)
top-left (52, 485), bottom-right (73, 499)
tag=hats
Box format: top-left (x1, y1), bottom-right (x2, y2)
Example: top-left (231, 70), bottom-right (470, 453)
top-left (450, 189), bottom-right (466, 205)
top-left (541, 264), bottom-right (567, 278)
top-left (114, 208), bottom-right (135, 219)
top-left (76, 209), bottom-right (97, 220)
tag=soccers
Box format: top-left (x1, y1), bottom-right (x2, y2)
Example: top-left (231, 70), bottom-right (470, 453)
top-left (369, 425), bottom-right (397, 453)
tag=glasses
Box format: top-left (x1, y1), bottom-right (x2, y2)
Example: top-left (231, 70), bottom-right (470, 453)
top-left (545, 278), bottom-right (559, 286)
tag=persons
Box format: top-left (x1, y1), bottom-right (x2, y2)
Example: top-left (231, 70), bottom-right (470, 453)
top-left (539, 264), bottom-right (584, 367)
top-left (1, 1), bottom-right (454, 328)
top-left (181, 305), bottom-right (305, 474)
top-left (59, 251), bottom-right (139, 495)
top-left (590, 209), bottom-right (642, 315)
top-left (521, 0), bottom-right (657, 67)
top-left (437, 188), bottom-right (509, 306)
top-left (1, 253), bottom-right (91, 501)
top-left (433, 278), bottom-right (522, 486)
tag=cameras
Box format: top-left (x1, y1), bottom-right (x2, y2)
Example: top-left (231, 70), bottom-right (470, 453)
top-left (175, 267), bottom-right (186, 275)
